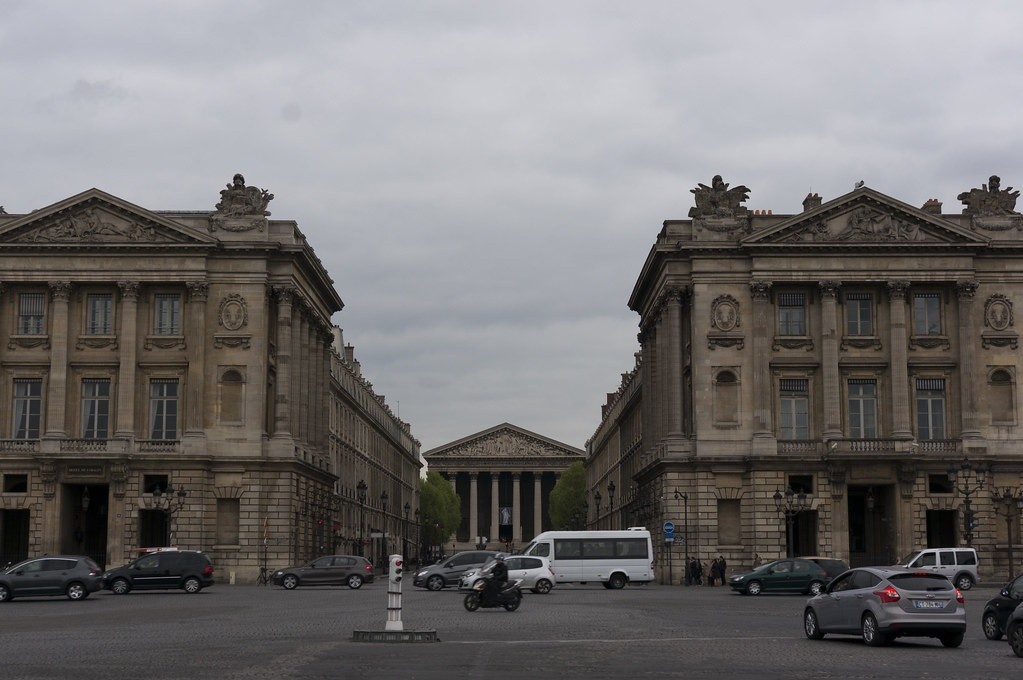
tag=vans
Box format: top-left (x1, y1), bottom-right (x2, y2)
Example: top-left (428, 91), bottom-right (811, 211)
top-left (893, 547), bottom-right (979, 590)
top-left (102, 549), bottom-right (214, 594)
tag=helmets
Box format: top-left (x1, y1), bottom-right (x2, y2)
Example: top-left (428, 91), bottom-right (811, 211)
top-left (495, 553), bottom-right (504, 559)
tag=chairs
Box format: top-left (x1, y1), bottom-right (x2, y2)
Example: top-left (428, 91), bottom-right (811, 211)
top-left (559, 543), bottom-right (601, 553)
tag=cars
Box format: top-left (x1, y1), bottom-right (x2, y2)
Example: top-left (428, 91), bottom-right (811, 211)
top-left (811, 558), bottom-right (851, 581)
top-left (728, 559), bottom-right (828, 596)
top-left (0, 552), bottom-right (104, 603)
top-left (413, 550), bottom-right (512, 590)
top-left (272, 554), bottom-right (375, 589)
top-left (803, 567), bottom-right (967, 648)
top-left (981, 573), bottom-right (1023, 658)
top-left (457, 555), bottom-right (556, 594)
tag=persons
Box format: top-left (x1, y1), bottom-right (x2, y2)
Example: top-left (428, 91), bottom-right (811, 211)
top-left (228, 173), bottom-right (247, 217)
top-left (754, 554), bottom-right (762, 568)
top-left (40, 553), bottom-right (48, 570)
top-left (74, 208), bottom-right (128, 237)
top-left (481, 553), bottom-right (508, 603)
top-left (130, 222), bottom-right (145, 240)
top-left (503, 538), bottom-right (514, 553)
top-left (817, 207), bottom-right (909, 241)
top-left (6, 562), bottom-right (12, 570)
top-left (686, 556), bottom-right (727, 587)
top-left (53, 219), bottom-right (66, 237)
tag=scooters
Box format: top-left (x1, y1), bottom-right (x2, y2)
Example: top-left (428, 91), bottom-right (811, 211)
top-left (463, 555), bottom-right (523, 612)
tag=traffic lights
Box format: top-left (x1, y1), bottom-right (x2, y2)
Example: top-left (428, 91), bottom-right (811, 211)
top-left (392, 558), bottom-right (404, 584)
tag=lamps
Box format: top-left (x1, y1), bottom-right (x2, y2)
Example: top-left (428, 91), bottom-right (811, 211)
top-left (83, 485), bottom-right (91, 511)
top-left (866, 489), bottom-right (875, 512)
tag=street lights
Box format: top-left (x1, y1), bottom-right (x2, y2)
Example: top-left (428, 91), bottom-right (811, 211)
top-left (380, 490), bottom-right (389, 573)
top-left (991, 485), bottom-right (1023, 582)
top-left (773, 483), bottom-right (807, 559)
top-left (594, 491), bottom-right (601, 530)
top-left (946, 454), bottom-right (986, 547)
top-left (673, 488), bottom-right (689, 585)
top-left (153, 481), bottom-right (186, 547)
top-left (414, 508), bottom-right (420, 570)
top-left (607, 481), bottom-right (615, 530)
top-left (357, 479), bottom-right (367, 556)
top-left (425, 513), bottom-right (437, 563)
top-left (565, 500), bottom-right (589, 529)
top-left (404, 502), bottom-right (410, 571)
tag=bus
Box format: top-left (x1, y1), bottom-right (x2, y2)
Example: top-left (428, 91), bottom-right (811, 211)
top-left (519, 527), bottom-right (655, 589)
